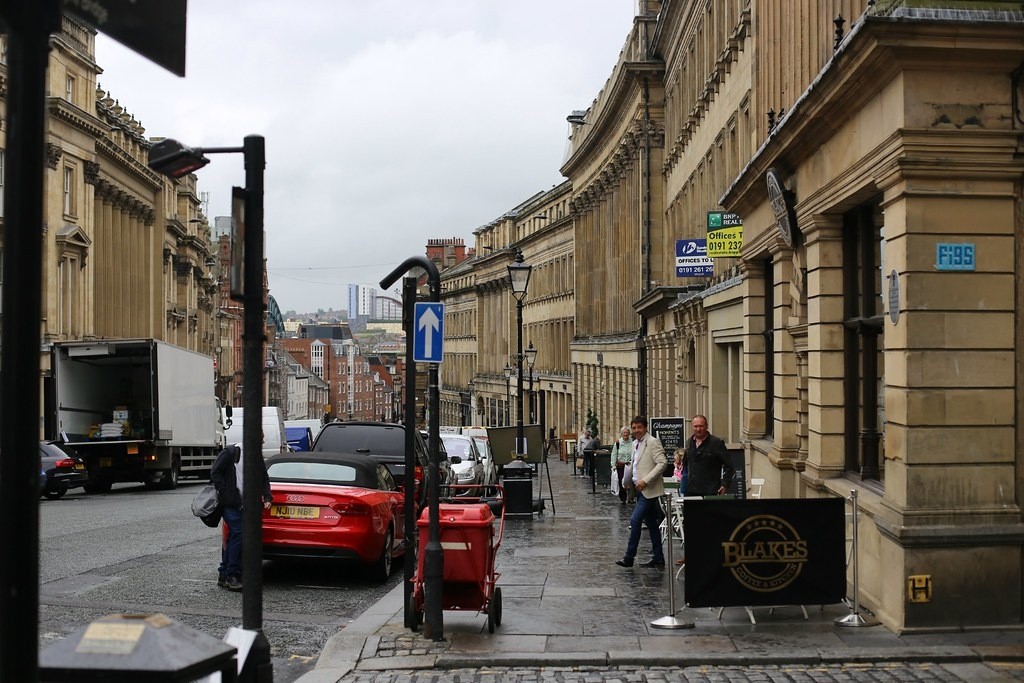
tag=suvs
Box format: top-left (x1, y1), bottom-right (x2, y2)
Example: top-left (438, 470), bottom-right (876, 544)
top-left (312, 415), bottom-right (430, 515)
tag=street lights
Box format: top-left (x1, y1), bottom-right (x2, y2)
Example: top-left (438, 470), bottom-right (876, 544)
top-left (147, 133), bottom-right (268, 636)
top-left (468, 246), bottom-right (552, 521)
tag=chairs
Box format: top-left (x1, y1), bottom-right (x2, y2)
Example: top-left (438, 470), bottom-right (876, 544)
top-left (655, 474), bottom-right (765, 588)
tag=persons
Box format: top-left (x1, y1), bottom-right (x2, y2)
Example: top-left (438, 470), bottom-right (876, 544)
top-left (577, 430), bottom-right (594, 479)
top-left (584, 436), bottom-right (602, 478)
top-left (674, 415), bottom-right (736, 565)
top-left (610, 426), bottom-right (637, 505)
top-left (546, 426), bottom-right (558, 456)
top-left (672, 447), bottom-right (687, 481)
top-left (616, 416), bottom-right (669, 567)
top-left (210, 428), bottom-right (274, 591)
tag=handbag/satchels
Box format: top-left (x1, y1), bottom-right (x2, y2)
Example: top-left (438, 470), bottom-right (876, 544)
top-left (610, 467), bottom-right (620, 496)
top-left (190, 482), bottom-right (224, 527)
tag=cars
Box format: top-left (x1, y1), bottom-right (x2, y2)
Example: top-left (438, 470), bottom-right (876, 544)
top-left (421, 431), bottom-right (500, 502)
top-left (40, 440), bottom-right (88, 501)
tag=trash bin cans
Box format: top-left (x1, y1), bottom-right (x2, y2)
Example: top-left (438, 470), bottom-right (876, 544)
top-left (416, 502), bottom-right (497, 584)
top-left (596, 445), bottom-right (613, 487)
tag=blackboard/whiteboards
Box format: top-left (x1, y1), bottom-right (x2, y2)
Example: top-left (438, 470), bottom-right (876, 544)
top-left (486, 423), bottom-right (545, 466)
top-left (650, 416), bottom-right (688, 478)
top-left (717, 441), bottom-right (752, 499)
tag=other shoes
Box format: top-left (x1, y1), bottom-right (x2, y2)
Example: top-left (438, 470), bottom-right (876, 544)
top-left (675, 558), bottom-right (685, 565)
top-left (224, 575), bottom-right (244, 589)
top-left (217, 575), bottom-right (229, 588)
top-left (640, 559), bottom-right (665, 567)
top-left (616, 559), bottom-right (633, 567)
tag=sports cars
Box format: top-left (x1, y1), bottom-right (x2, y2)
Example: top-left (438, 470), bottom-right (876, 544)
top-left (222, 451), bottom-right (421, 585)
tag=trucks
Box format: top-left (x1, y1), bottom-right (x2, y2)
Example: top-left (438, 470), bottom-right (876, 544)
top-left (52, 338), bottom-right (234, 493)
top-left (221, 406), bottom-right (320, 462)
